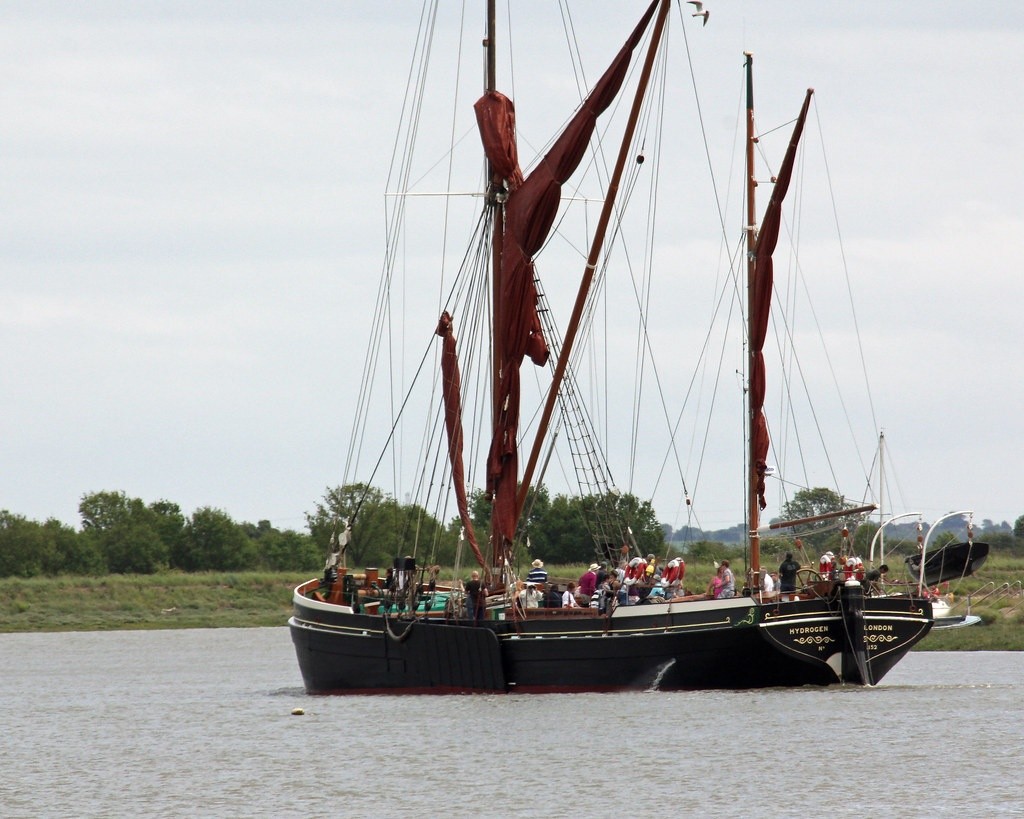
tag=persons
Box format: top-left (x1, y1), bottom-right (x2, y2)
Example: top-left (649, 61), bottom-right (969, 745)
top-left (819, 550), bottom-right (889, 597)
top-left (515, 554), bottom-right (694, 616)
top-left (465, 570), bottom-right (489, 620)
top-left (759, 551), bottom-right (801, 592)
top-left (384, 567), bottom-right (393, 589)
top-left (704, 560), bottom-right (735, 600)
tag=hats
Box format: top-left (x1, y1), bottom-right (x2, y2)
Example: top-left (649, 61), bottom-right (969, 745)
top-left (588, 563), bottom-right (602, 571)
top-left (527, 582), bottom-right (535, 586)
top-left (531, 559), bottom-right (543, 568)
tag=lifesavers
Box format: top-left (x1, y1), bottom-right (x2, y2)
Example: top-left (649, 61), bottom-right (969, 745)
top-left (843, 557), bottom-right (864, 582)
top-left (923, 585), bottom-right (940, 602)
top-left (631, 558), bottom-right (648, 586)
top-left (819, 551), bottom-right (836, 581)
top-left (673, 557), bottom-right (685, 581)
top-left (660, 560), bottom-right (680, 588)
top-left (624, 557), bottom-right (645, 586)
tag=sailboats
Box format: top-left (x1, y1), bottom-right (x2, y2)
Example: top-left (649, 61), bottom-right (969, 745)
top-left (286, 0), bottom-right (934, 696)
top-left (857, 511), bottom-right (981, 631)
